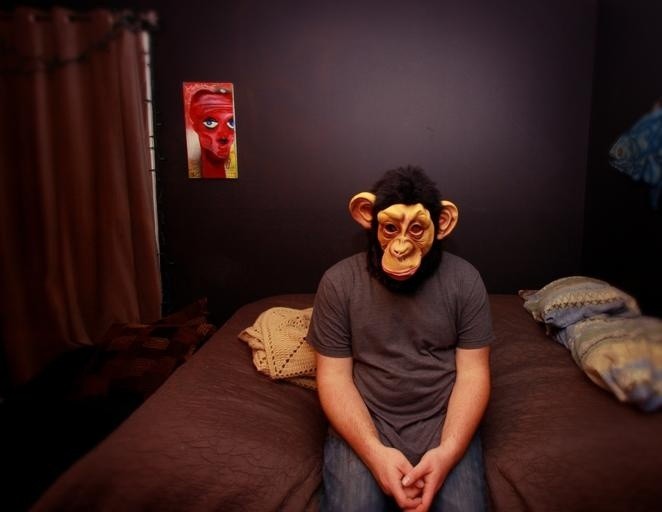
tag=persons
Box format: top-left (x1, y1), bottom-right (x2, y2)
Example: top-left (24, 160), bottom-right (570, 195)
top-left (189, 88), bottom-right (241, 182)
top-left (302, 162), bottom-right (503, 511)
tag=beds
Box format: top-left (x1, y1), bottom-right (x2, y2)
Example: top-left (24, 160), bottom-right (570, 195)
top-left (25, 291), bottom-right (661, 512)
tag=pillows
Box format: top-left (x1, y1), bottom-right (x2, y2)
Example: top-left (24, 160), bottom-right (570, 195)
top-left (519, 275), bottom-right (662, 411)
